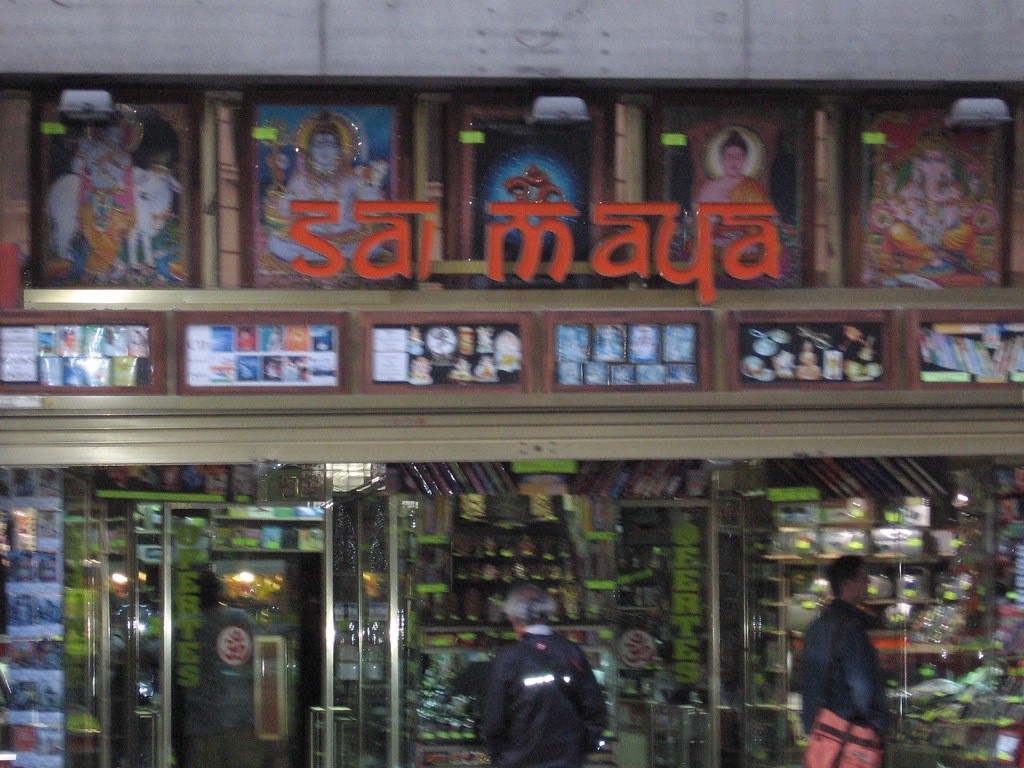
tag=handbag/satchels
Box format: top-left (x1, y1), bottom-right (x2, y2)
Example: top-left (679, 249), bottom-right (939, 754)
top-left (804, 707), bottom-right (883, 768)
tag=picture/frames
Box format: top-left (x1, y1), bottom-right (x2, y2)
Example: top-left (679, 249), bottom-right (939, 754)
top-left (32, 91), bottom-right (203, 290)
top-left (174, 310), bottom-right (352, 393)
top-left (724, 308), bottom-right (900, 391)
top-left (845, 90), bottom-right (1013, 289)
top-left (248, 95), bottom-right (412, 292)
top-left (461, 105), bottom-right (606, 289)
top-left (0, 311), bottom-right (167, 396)
top-left (359, 311), bottom-right (533, 394)
top-left (903, 308), bottom-right (1024, 390)
top-left (543, 309), bottom-right (718, 392)
top-left (645, 92), bottom-right (815, 289)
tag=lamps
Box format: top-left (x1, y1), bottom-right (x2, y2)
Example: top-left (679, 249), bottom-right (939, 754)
top-left (944, 96), bottom-right (1013, 129)
top-left (53, 89), bottom-right (116, 122)
top-left (526, 95), bottom-right (592, 127)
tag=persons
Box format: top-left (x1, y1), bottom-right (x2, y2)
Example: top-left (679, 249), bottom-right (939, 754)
top-left (68, 111), bottom-right (779, 279)
top-left (481, 584), bottom-right (609, 768)
top-left (801, 554), bottom-right (898, 768)
